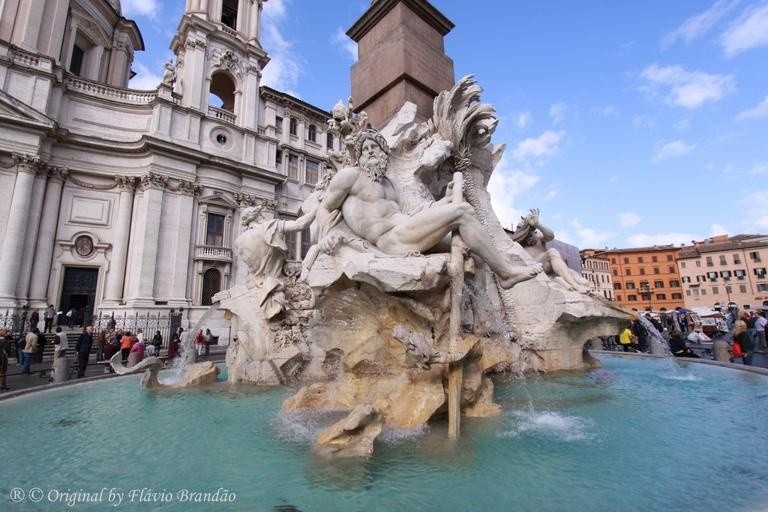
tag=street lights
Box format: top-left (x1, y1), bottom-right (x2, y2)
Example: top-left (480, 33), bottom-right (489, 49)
top-left (645, 282), bottom-right (652, 311)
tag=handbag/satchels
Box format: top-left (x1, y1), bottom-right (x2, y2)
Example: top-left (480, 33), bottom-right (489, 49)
top-left (17, 337), bottom-right (26, 349)
top-left (733, 343), bottom-right (742, 357)
top-left (53, 335), bottom-right (61, 343)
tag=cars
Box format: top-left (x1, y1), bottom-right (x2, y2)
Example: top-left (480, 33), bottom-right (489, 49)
top-left (692, 307), bottom-right (722, 335)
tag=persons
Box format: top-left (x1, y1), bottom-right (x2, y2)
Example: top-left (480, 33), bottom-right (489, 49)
top-left (0, 305), bottom-right (213, 392)
top-left (316, 126), bottom-right (544, 289)
top-left (599, 310), bottom-right (768, 366)
top-left (235, 204), bottom-right (317, 319)
top-left (516, 208), bottom-right (590, 294)
